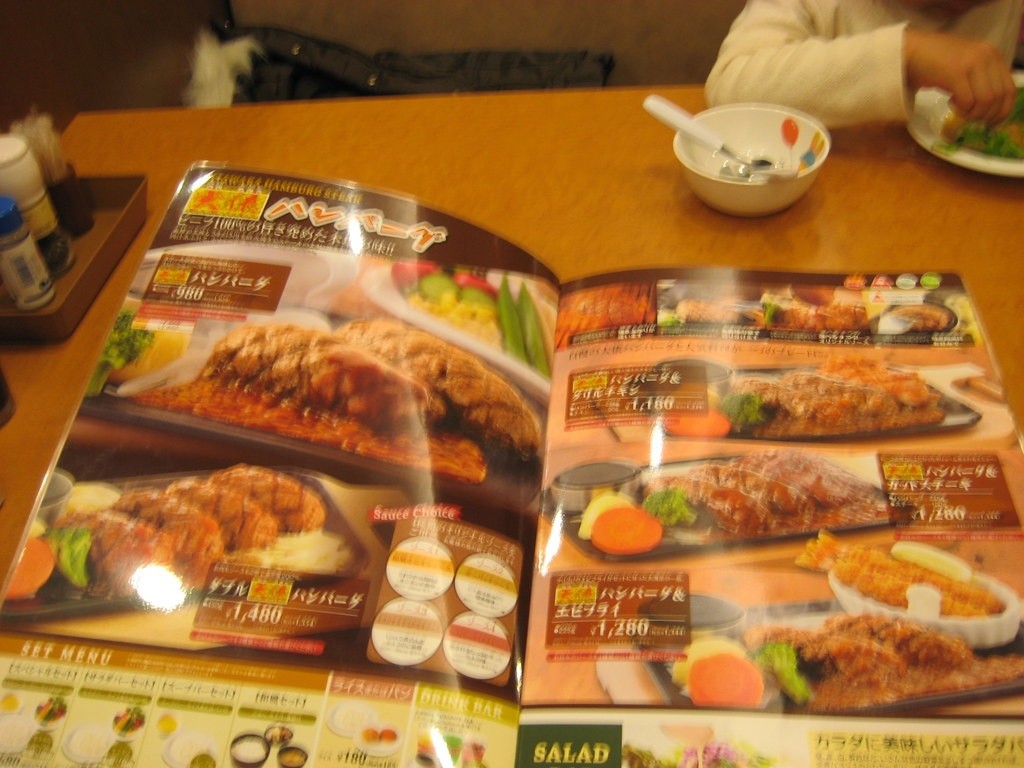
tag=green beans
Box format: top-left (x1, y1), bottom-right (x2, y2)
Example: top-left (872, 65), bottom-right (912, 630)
top-left (496, 275), bottom-right (551, 378)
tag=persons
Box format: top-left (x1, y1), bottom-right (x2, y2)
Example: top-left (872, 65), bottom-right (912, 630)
top-left (702, 0), bottom-right (1024, 129)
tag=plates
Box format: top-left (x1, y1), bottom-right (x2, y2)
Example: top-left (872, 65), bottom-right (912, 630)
top-left (33, 700), bottom-right (69, 730)
top-left (627, 598), bottom-right (1024, 714)
top-left (540, 453), bottom-right (918, 560)
top-left (110, 714), bottom-right (146, 742)
top-left (0, 464), bottom-right (371, 625)
top-left (78, 399), bottom-right (548, 517)
top-left (906, 70), bottom-right (1024, 178)
top-left (630, 364), bottom-right (982, 440)
top-left (62, 723), bottom-right (114, 763)
top-left (327, 700), bottom-right (377, 738)
top-left (161, 731), bottom-right (216, 768)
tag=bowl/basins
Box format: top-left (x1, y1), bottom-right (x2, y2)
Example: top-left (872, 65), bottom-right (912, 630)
top-left (353, 723), bottom-right (403, 757)
top-left (358, 261), bottom-right (557, 407)
top-left (230, 733), bottom-right (271, 768)
top-left (672, 103), bottom-right (832, 218)
top-left (0, 715), bottom-right (34, 754)
top-left (278, 747), bottom-right (308, 768)
top-left (827, 566), bottom-right (1021, 649)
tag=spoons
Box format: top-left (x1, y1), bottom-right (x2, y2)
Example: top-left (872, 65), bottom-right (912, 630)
top-left (643, 94), bottom-right (772, 177)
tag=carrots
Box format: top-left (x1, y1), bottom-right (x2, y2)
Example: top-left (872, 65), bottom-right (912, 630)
top-left (6, 538), bottom-right (56, 600)
top-left (664, 410), bottom-right (732, 439)
top-left (590, 508), bottom-right (663, 555)
top-left (688, 654), bottom-right (765, 709)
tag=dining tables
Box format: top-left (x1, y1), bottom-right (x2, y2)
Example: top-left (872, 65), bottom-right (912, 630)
top-left (1, 82), bottom-right (1023, 598)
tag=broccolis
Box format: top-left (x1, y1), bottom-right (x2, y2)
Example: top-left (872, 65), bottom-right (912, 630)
top-left (760, 301), bottom-right (783, 323)
top-left (641, 484), bottom-right (698, 528)
top-left (41, 527), bottom-right (93, 588)
top-left (751, 641), bottom-right (811, 707)
top-left (720, 390), bottom-right (769, 432)
top-left (82, 310), bottom-right (154, 397)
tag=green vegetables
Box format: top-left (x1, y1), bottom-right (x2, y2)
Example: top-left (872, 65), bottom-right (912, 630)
top-left (929, 85), bottom-right (1024, 160)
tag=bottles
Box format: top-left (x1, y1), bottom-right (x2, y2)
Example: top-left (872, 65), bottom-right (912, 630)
top-left (0, 135), bottom-right (75, 275)
top-left (0, 195), bottom-right (56, 312)
